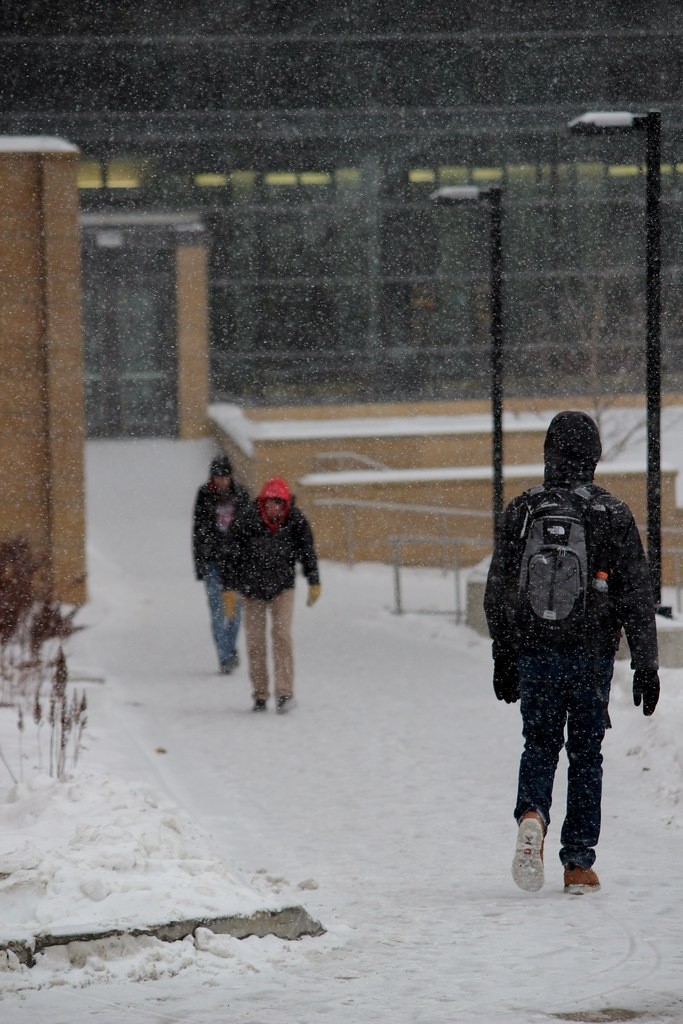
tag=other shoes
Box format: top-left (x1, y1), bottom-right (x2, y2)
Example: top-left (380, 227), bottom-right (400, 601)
top-left (276, 694), bottom-right (295, 712)
top-left (230, 650), bottom-right (240, 668)
top-left (221, 660), bottom-right (234, 673)
top-left (254, 697), bottom-right (268, 712)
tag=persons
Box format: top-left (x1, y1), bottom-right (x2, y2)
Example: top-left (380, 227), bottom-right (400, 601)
top-left (221, 475), bottom-right (323, 716)
top-left (483, 411), bottom-right (662, 900)
top-left (189, 452), bottom-right (248, 674)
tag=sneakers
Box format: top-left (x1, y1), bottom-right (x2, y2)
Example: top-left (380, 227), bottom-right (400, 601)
top-left (512, 811), bottom-right (547, 892)
top-left (563, 866), bottom-right (601, 895)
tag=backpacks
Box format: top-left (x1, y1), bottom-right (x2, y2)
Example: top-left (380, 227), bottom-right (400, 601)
top-left (521, 485), bottom-right (606, 644)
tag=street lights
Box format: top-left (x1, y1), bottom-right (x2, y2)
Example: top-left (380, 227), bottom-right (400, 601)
top-left (425, 182), bottom-right (506, 541)
top-left (568, 108), bottom-right (663, 609)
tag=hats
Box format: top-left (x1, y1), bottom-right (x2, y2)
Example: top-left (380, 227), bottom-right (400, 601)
top-left (211, 455), bottom-right (232, 478)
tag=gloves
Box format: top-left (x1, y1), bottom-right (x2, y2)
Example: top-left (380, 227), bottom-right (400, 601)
top-left (491, 660), bottom-right (523, 704)
top-left (221, 592), bottom-right (237, 617)
top-left (308, 584), bottom-right (321, 606)
top-left (632, 669), bottom-right (660, 717)
